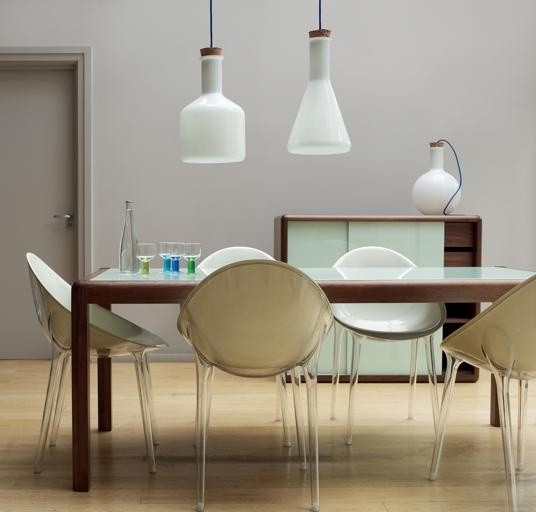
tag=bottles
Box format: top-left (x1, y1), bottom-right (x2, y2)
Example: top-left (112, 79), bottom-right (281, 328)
top-left (119, 201), bottom-right (139, 274)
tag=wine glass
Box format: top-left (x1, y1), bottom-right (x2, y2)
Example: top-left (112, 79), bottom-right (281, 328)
top-left (136, 242), bottom-right (201, 277)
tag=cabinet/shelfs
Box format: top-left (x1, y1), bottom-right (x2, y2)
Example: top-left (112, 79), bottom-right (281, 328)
top-left (273, 215), bottom-right (482, 384)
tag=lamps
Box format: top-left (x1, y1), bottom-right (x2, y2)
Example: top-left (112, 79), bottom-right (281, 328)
top-left (179, 0), bottom-right (247, 164)
top-left (287, 1), bottom-right (352, 155)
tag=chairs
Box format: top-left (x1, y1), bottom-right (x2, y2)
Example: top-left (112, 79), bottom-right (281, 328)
top-left (176, 259), bottom-right (334, 512)
top-left (26, 252), bottom-right (172, 475)
top-left (427, 276), bottom-right (536, 512)
top-left (71, 266), bottom-right (536, 493)
top-left (190, 247), bottom-right (291, 446)
top-left (328, 246), bottom-right (447, 446)
